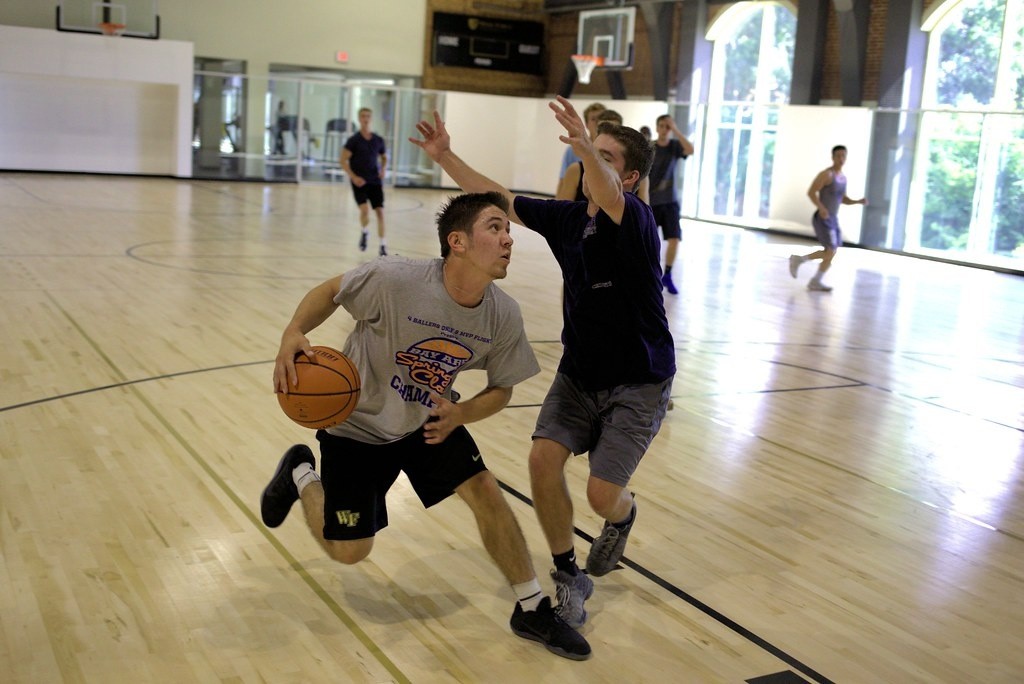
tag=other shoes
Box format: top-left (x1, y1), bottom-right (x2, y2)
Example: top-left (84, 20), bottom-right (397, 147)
top-left (359, 232), bottom-right (370, 251)
top-left (661, 275), bottom-right (678, 294)
top-left (380, 246), bottom-right (388, 256)
top-left (789, 255), bottom-right (802, 278)
top-left (808, 278), bottom-right (832, 290)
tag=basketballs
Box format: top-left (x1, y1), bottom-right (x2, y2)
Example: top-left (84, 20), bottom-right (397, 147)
top-left (277, 344), bottom-right (362, 430)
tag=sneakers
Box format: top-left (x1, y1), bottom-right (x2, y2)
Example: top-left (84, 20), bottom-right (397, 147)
top-left (550, 570), bottom-right (594, 629)
top-left (586, 492), bottom-right (637, 577)
top-left (260, 444), bottom-right (315, 528)
top-left (510, 596), bottom-right (592, 660)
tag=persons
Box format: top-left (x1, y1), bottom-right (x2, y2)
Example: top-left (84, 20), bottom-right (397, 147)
top-left (789, 146), bottom-right (870, 292)
top-left (259, 191), bottom-right (596, 662)
top-left (271, 99), bottom-right (291, 156)
top-left (339, 108), bottom-right (389, 258)
top-left (409, 75), bottom-right (696, 631)
